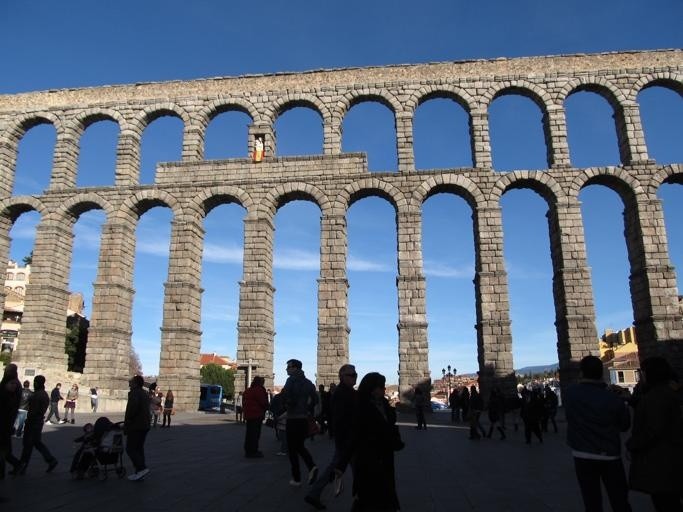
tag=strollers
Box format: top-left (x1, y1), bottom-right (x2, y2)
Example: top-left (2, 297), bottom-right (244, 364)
top-left (70, 417), bottom-right (128, 480)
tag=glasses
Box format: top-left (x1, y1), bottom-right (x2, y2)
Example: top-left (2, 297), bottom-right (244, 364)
top-left (344, 373), bottom-right (356, 378)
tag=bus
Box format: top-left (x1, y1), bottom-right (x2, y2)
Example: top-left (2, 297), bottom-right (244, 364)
top-left (199, 384), bottom-right (223, 409)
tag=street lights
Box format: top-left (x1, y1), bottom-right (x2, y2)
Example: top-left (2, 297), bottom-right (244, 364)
top-left (442, 365), bottom-right (457, 407)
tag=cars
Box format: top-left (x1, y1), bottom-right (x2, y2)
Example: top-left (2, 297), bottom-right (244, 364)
top-left (430, 400), bottom-right (448, 410)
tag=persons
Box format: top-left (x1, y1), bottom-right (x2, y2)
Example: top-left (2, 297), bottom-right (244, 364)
top-left (121, 375), bottom-right (174, 481)
top-left (561, 356), bottom-right (683, 511)
top-left (449, 379), bottom-right (559, 448)
top-left (0, 363), bottom-right (98, 476)
top-left (235, 359), bottom-right (405, 512)
top-left (412, 387), bottom-right (427, 431)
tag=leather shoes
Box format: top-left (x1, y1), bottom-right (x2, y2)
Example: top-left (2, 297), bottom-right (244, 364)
top-left (46, 459), bottom-right (57, 473)
top-left (304, 496), bottom-right (327, 510)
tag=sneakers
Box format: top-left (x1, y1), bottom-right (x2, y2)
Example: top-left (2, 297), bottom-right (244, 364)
top-left (127, 469), bottom-right (149, 481)
top-left (306, 465), bottom-right (318, 486)
top-left (289, 480), bottom-right (302, 487)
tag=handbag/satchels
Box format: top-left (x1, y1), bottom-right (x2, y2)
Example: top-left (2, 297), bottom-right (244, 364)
top-left (307, 415), bottom-right (319, 436)
